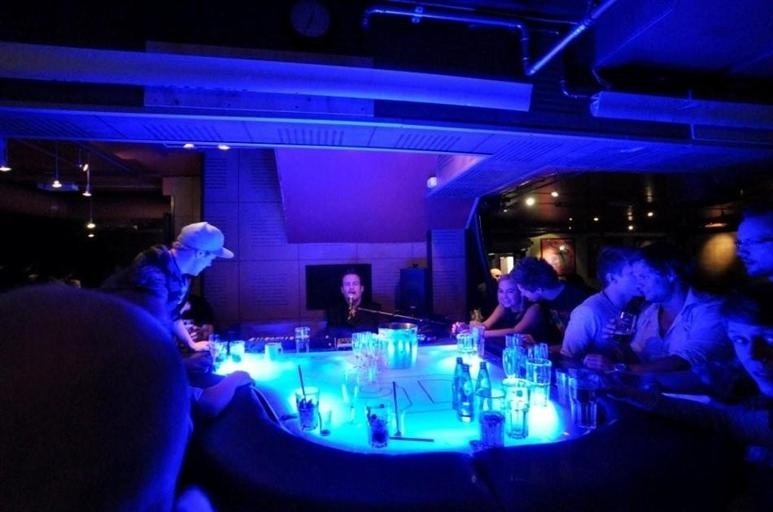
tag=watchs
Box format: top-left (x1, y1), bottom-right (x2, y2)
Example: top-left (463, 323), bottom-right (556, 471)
top-left (609, 360), bottom-right (629, 376)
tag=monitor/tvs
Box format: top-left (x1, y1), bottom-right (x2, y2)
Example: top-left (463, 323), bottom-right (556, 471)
top-left (306, 263), bottom-right (372, 311)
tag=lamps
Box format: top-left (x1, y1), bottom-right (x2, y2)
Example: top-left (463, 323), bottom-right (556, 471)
top-left (86, 221), bottom-right (96, 228)
top-left (52, 180), bottom-right (62, 188)
top-left (0, 165), bottom-right (12, 173)
top-left (82, 190), bottom-right (92, 197)
top-left (78, 162), bottom-right (90, 172)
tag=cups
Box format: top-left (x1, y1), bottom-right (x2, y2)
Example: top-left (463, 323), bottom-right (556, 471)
top-left (524, 358), bottom-right (553, 385)
top-left (502, 385), bottom-right (532, 440)
top-left (473, 385), bottom-right (507, 449)
top-left (228, 340), bottom-right (246, 365)
top-left (502, 348), bottom-right (523, 387)
top-left (215, 341), bottom-right (228, 365)
top-left (208, 333), bottom-right (220, 369)
top-left (505, 332), bottom-right (524, 346)
top-left (554, 367), bottom-right (567, 406)
top-left (264, 341), bottom-right (285, 363)
top-left (527, 345), bottom-right (534, 359)
top-left (472, 326), bottom-right (485, 357)
top-left (455, 332), bottom-right (474, 352)
top-left (365, 402), bottom-right (390, 450)
top-left (294, 325), bottom-right (311, 359)
top-left (568, 368), bottom-right (601, 432)
top-left (295, 386), bottom-right (321, 434)
top-left (533, 341), bottom-right (550, 358)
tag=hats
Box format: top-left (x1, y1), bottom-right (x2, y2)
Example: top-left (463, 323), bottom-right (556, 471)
top-left (178, 222), bottom-right (233, 259)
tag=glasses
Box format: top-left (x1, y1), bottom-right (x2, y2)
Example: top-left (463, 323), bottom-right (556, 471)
top-left (734, 235), bottom-right (773, 247)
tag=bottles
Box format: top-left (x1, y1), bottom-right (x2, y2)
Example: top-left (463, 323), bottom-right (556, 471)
top-left (457, 365), bottom-right (474, 422)
top-left (476, 362), bottom-right (491, 412)
top-left (452, 357), bottom-right (463, 410)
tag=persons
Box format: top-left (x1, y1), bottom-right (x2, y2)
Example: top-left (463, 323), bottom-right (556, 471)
top-left (179, 294), bottom-right (218, 342)
top-left (101, 222), bottom-right (235, 359)
top-left (0, 286), bottom-right (213, 512)
top-left (509, 256), bottom-right (594, 354)
top-left (720, 281), bottom-right (773, 461)
top-left (583, 245), bottom-right (733, 374)
top-left (560, 251), bottom-right (641, 363)
top-left (735, 210), bottom-right (773, 280)
top-left (322, 272), bottom-right (382, 331)
top-left (450, 275), bottom-right (541, 342)
